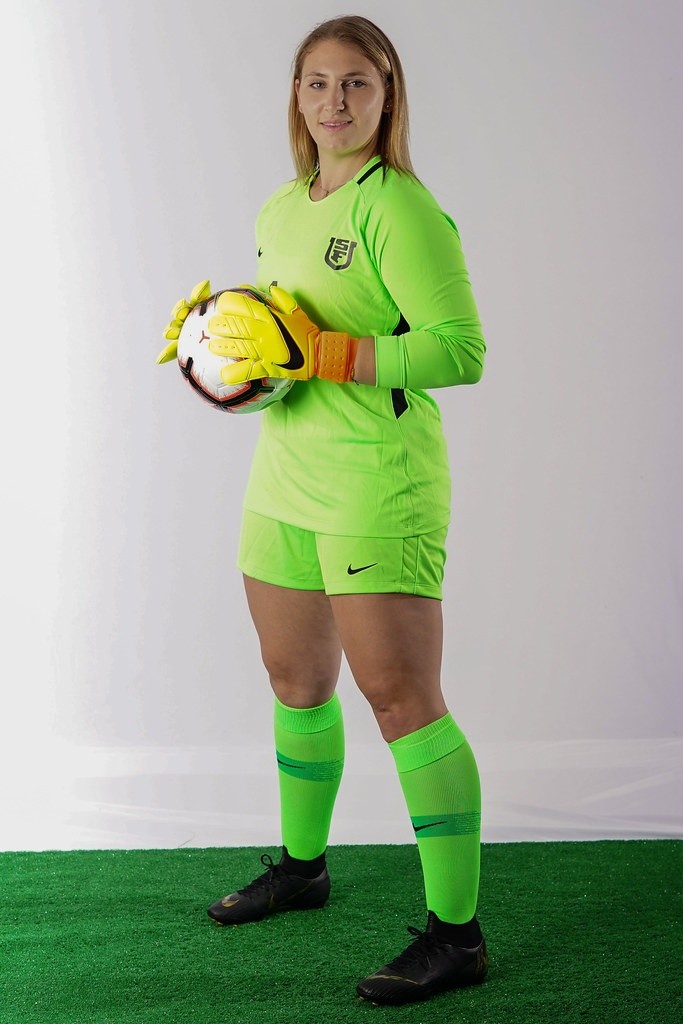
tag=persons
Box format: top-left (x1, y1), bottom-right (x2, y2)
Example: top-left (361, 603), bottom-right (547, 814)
top-left (156, 15), bottom-right (487, 1007)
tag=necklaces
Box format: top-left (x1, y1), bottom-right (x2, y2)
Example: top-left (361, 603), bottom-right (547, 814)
top-left (316, 182), bottom-right (348, 199)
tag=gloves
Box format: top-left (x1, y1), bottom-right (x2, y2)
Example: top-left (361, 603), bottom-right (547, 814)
top-left (207, 285), bottom-right (352, 389)
top-left (156, 281), bottom-right (212, 365)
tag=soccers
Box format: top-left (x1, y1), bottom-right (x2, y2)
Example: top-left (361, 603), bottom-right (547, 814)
top-left (178, 288), bottom-right (297, 415)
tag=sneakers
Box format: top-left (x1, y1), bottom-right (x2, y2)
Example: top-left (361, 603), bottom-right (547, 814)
top-left (356, 931), bottom-right (487, 1005)
top-left (206, 865), bottom-right (331, 924)
